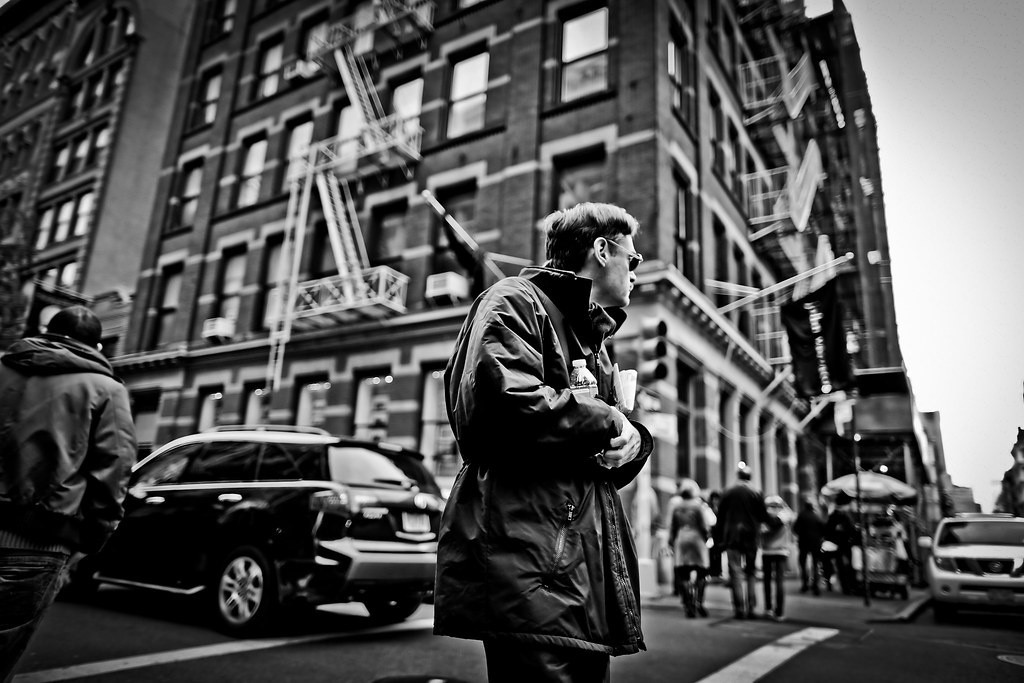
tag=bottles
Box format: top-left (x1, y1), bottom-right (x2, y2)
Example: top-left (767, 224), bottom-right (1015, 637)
top-left (569, 358), bottom-right (599, 400)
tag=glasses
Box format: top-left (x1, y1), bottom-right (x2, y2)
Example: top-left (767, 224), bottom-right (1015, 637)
top-left (605, 238), bottom-right (643, 271)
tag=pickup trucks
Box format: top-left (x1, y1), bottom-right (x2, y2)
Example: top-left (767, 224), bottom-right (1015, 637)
top-left (917, 513), bottom-right (1024, 629)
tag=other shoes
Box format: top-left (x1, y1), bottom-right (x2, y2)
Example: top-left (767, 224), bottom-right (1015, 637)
top-left (694, 601), bottom-right (709, 619)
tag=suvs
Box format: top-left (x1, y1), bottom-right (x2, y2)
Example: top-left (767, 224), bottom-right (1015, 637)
top-left (50, 423), bottom-right (450, 639)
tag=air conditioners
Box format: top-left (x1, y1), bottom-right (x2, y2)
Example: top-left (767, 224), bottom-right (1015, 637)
top-left (200, 316), bottom-right (237, 345)
top-left (423, 272), bottom-right (470, 308)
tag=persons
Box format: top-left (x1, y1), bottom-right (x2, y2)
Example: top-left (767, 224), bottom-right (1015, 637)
top-left (873, 512), bottom-right (909, 579)
top-left (792, 502), bottom-right (853, 598)
top-left (434, 201), bottom-right (655, 683)
top-left (762, 496), bottom-right (796, 621)
top-left (0, 305), bottom-right (137, 680)
top-left (717, 465), bottom-right (783, 620)
top-left (667, 478), bottom-right (722, 618)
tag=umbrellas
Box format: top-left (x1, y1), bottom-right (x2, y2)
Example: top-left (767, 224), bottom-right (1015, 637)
top-left (821, 472), bottom-right (917, 505)
